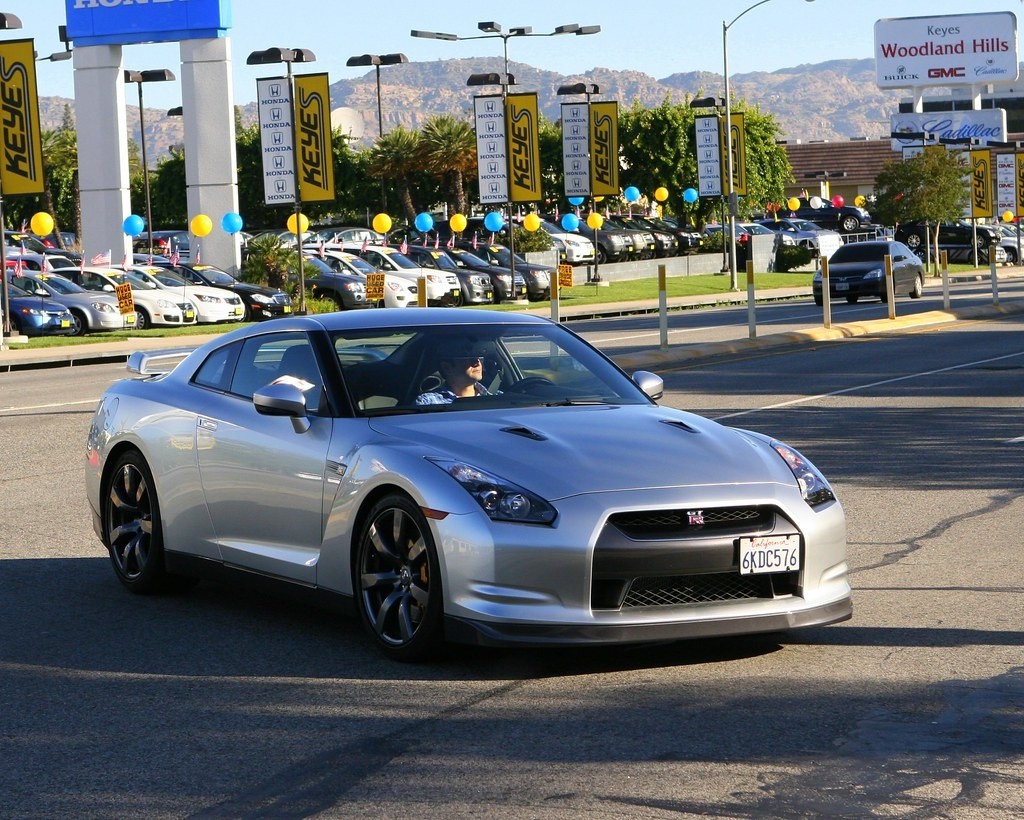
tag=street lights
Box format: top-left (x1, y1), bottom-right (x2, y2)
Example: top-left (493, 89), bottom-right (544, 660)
top-left (125, 70), bottom-right (175, 263)
top-left (723, 0), bottom-right (817, 292)
top-left (804, 170), bottom-right (846, 199)
top-left (346, 21), bottom-right (602, 215)
top-left (0, 12), bottom-right (71, 337)
top-left (247, 47), bottom-right (317, 314)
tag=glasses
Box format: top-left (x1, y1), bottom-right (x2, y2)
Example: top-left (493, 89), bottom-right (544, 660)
top-left (463, 359), bottom-right (483, 367)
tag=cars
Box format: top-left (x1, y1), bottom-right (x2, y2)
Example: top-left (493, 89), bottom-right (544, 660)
top-left (813, 241), bottom-right (925, 306)
top-left (895, 217), bottom-right (1024, 265)
top-left (1, 281), bottom-right (76, 336)
top-left (497, 218), bottom-right (844, 265)
top-left (134, 260), bottom-right (293, 321)
top-left (3, 230), bottom-right (191, 272)
top-left (768, 197), bottom-right (870, 232)
top-left (238, 227), bottom-right (563, 311)
top-left (101, 264), bottom-right (246, 324)
top-left (5, 270), bottom-right (138, 336)
top-left (48, 266), bottom-right (198, 330)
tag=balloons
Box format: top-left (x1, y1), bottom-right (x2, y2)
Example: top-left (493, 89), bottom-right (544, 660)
top-left (1003, 211), bottom-right (1014, 223)
top-left (524, 214), bottom-right (541, 231)
top-left (561, 213), bottom-right (579, 231)
top-left (855, 196), bottom-right (866, 207)
top-left (450, 214), bottom-right (468, 232)
top-left (655, 187), bottom-right (669, 202)
top-left (831, 195), bottom-right (844, 207)
top-left (810, 197), bottom-right (823, 210)
top-left (626, 187), bottom-right (639, 202)
top-left (32, 212), bottom-right (55, 237)
top-left (485, 213), bottom-right (502, 232)
top-left (683, 188), bottom-right (699, 203)
top-left (287, 212), bottom-right (309, 234)
top-left (415, 214), bottom-right (434, 233)
top-left (587, 212), bottom-right (603, 230)
top-left (787, 198), bottom-right (802, 210)
top-left (372, 214), bottom-right (392, 233)
top-left (122, 214), bottom-right (143, 237)
top-left (221, 212), bottom-right (243, 235)
top-left (568, 195), bottom-right (604, 207)
top-left (191, 214), bottom-right (213, 236)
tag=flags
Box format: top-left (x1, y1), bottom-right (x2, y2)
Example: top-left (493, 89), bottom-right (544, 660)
top-left (13, 237), bottom-right (201, 278)
top-left (502, 206), bottom-right (649, 221)
top-left (317, 231), bottom-right (495, 258)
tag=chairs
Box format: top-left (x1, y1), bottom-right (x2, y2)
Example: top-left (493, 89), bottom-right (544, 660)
top-left (269, 344), bottom-right (326, 412)
top-left (404, 343), bottom-right (449, 405)
top-left (250, 359), bottom-right (402, 409)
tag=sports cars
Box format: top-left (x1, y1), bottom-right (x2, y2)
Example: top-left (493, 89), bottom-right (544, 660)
top-left (85, 307), bottom-right (854, 663)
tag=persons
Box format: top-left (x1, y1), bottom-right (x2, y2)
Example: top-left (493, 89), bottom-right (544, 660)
top-left (409, 333), bottom-right (504, 410)
